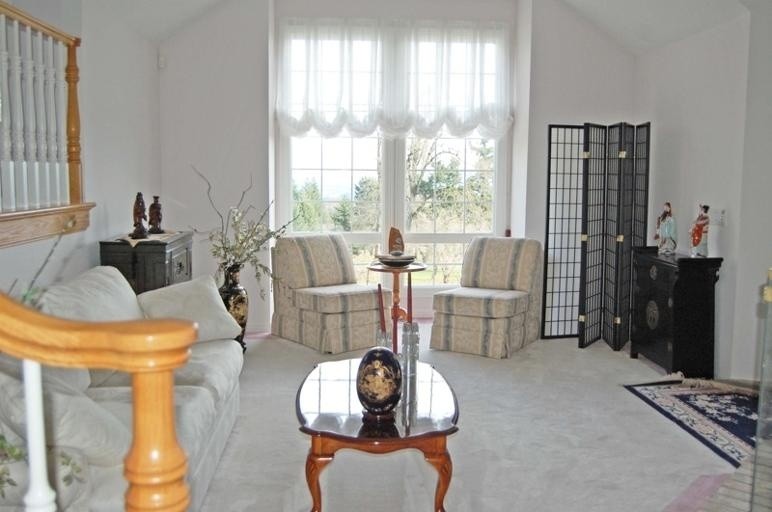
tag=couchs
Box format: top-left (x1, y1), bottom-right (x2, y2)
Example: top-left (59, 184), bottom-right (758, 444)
top-left (270, 232), bottom-right (393, 355)
top-left (428, 236), bottom-right (545, 359)
top-left (0, 299), bottom-right (244, 512)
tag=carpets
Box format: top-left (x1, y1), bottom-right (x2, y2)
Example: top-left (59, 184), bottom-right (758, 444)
top-left (620, 381), bottom-right (772, 468)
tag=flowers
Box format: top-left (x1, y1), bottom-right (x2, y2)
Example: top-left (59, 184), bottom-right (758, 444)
top-left (194, 165), bottom-right (292, 301)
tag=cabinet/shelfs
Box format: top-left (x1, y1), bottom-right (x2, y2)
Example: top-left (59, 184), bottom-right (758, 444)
top-left (628, 245), bottom-right (724, 379)
top-left (99, 231), bottom-right (195, 295)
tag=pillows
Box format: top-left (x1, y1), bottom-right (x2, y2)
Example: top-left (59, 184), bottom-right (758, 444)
top-left (4, 365), bottom-right (132, 466)
top-left (138, 276), bottom-right (242, 342)
top-left (41, 265), bottom-right (146, 386)
top-left (0, 349), bottom-right (93, 390)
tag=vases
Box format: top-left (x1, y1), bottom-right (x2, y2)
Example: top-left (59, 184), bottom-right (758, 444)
top-left (218, 262), bottom-right (249, 354)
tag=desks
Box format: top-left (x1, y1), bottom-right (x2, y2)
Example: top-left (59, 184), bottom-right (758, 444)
top-left (366, 258), bottom-right (427, 354)
